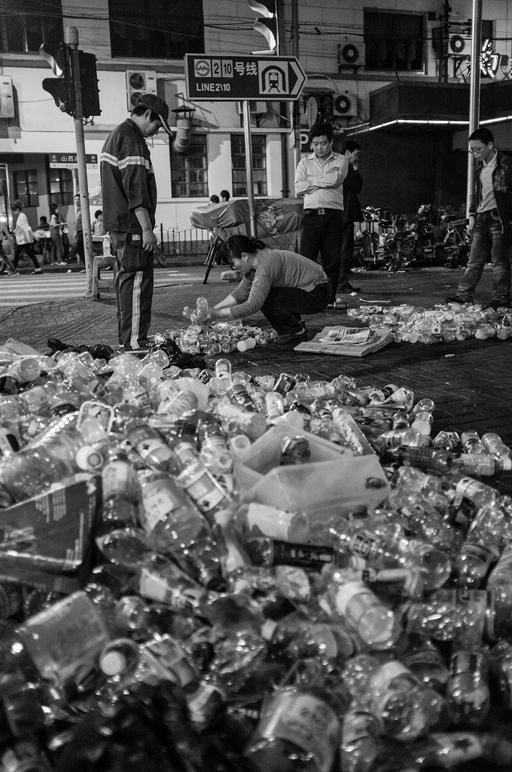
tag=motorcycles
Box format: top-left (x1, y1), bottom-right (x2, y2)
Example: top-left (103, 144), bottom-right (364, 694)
top-left (0, 230), bottom-right (30, 275)
top-left (356, 202), bottom-right (467, 274)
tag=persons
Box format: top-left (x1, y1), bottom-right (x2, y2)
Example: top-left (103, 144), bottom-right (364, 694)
top-left (336, 140), bottom-right (365, 293)
top-left (100, 94), bottom-right (174, 354)
top-left (294, 122), bottom-right (349, 309)
top-left (189, 235), bottom-right (329, 345)
top-left (445, 127), bottom-right (512, 311)
top-left (0, 194), bottom-right (112, 278)
top-left (209, 195), bottom-right (219, 205)
top-left (219, 190), bottom-right (230, 202)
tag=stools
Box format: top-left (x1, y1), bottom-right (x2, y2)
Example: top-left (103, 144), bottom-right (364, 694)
top-left (91, 255), bottom-right (119, 301)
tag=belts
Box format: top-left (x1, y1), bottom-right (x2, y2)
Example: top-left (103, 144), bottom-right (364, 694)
top-left (304, 208), bottom-right (343, 216)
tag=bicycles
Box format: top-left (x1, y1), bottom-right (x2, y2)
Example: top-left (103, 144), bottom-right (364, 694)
top-left (201, 232), bottom-right (235, 284)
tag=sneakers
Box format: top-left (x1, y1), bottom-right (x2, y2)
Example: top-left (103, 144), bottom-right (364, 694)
top-left (118, 342), bottom-right (159, 354)
top-left (485, 301), bottom-right (508, 310)
top-left (31, 269), bottom-right (43, 275)
top-left (336, 282), bottom-right (362, 295)
top-left (5, 268), bottom-right (20, 279)
top-left (273, 321), bottom-right (306, 344)
top-left (55, 261), bottom-right (68, 266)
top-left (446, 296), bottom-right (469, 304)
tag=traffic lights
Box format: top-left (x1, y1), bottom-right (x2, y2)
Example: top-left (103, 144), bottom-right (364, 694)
top-left (38, 41), bottom-right (75, 118)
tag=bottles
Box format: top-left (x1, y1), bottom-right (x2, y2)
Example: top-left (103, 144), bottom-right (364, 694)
top-left (348, 298), bottom-right (510, 341)
top-left (157, 295), bottom-right (282, 357)
top-left (2, 341), bottom-right (512, 772)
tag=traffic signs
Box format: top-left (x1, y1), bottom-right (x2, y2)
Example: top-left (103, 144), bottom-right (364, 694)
top-left (183, 52), bottom-right (306, 102)
top-left (48, 152), bottom-right (98, 171)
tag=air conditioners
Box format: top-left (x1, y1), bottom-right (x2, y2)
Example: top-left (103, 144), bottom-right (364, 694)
top-left (336, 41), bottom-right (367, 66)
top-left (235, 100), bottom-right (267, 115)
top-left (124, 70), bottom-right (158, 113)
top-left (332, 94), bottom-right (358, 116)
top-left (0, 74), bottom-right (16, 119)
top-left (443, 33), bottom-right (472, 57)
